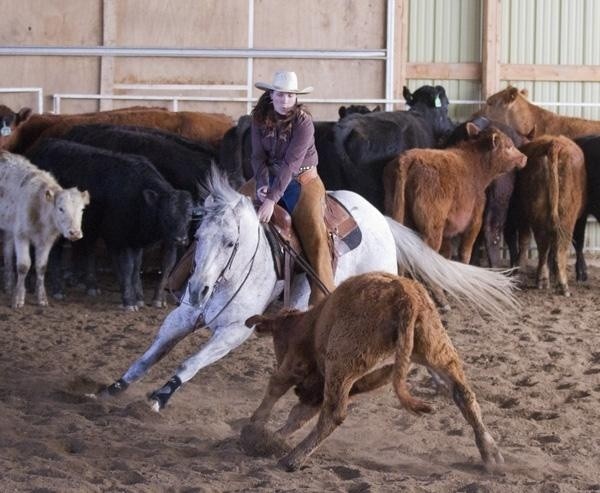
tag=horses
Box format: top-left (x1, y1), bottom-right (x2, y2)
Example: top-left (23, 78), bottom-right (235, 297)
top-left (98, 158), bottom-right (527, 418)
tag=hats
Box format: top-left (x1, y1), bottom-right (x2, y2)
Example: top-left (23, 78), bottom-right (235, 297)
top-left (255, 71), bottom-right (314, 94)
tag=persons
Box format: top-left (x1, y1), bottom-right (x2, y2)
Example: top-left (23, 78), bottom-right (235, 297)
top-left (162, 69), bottom-right (337, 310)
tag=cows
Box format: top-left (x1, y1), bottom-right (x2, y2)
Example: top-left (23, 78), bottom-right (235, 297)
top-left (0, 85), bottom-right (600, 310)
top-left (244, 271), bottom-right (504, 471)
